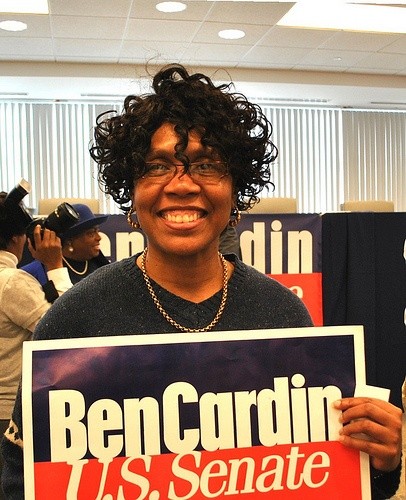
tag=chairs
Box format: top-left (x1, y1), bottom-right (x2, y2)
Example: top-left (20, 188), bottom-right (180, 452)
top-left (339, 201), bottom-right (394, 212)
top-left (242, 198), bottom-right (297, 213)
top-left (38, 198), bottom-right (99, 215)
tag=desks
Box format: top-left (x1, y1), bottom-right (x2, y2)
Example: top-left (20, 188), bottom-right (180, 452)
top-left (33, 213), bottom-right (406, 413)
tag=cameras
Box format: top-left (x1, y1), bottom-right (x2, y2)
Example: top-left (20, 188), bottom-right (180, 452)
top-left (25, 202), bottom-right (79, 248)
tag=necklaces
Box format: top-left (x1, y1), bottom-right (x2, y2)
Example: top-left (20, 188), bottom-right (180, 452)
top-left (142, 246), bottom-right (228, 332)
top-left (61, 256), bottom-right (88, 275)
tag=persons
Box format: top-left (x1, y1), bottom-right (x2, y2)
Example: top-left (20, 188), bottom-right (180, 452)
top-left (0, 191), bottom-right (74, 500)
top-left (0, 63), bottom-right (403, 500)
top-left (20, 204), bottom-right (109, 304)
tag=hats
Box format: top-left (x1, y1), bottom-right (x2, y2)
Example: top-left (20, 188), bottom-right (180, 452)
top-left (56, 203), bottom-right (109, 241)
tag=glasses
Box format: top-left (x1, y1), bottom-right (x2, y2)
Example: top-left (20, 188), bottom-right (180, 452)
top-left (142, 162), bottom-right (230, 182)
